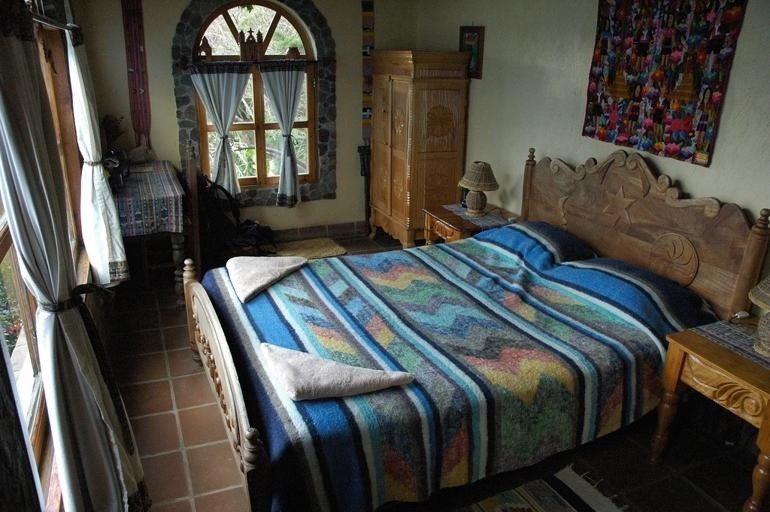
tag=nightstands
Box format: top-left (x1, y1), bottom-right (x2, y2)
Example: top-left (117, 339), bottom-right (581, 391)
top-left (423, 202), bottom-right (521, 247)
top-left (650, 312), bottom-right (770, 512)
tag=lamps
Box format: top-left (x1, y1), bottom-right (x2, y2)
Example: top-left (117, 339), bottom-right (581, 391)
top-left (747, 277), bottom-right (770, 358)
top-left (457, 160), bottom-right (500, 218)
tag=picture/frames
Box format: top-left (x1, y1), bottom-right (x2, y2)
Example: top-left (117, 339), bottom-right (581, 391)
top-left (459, 26), bottom-right (485, 80)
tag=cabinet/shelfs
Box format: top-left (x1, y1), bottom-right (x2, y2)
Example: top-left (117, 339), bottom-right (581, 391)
top-left (368, 49), bottom-right (469, 249)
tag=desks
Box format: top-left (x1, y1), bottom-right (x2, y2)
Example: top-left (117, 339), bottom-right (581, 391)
top-left (113, 161), bottom-right (186, 312)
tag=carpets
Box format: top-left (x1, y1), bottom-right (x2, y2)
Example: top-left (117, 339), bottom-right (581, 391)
top-left (242, 238), bottom-right (347, 258)
top-left (454, 457), bottom-right (640, 512)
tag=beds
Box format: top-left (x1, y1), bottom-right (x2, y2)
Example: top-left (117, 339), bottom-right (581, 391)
top-left (183, 149), bottom-right (770, 512)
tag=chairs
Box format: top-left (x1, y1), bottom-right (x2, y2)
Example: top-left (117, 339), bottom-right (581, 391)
top-left (140, 139), bottom-right (202, 290)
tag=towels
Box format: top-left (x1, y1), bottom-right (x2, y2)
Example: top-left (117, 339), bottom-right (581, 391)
top-left (260, 342), bottom-right (415, 402)
top-left (226, 255), bottom-right (308, 304)
